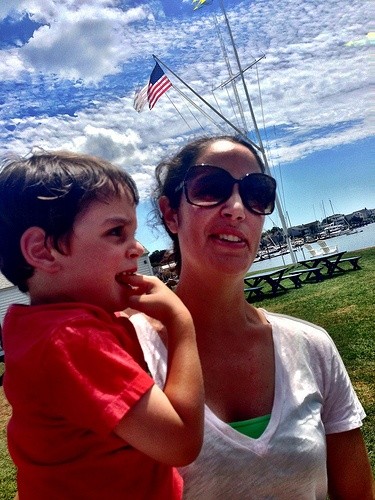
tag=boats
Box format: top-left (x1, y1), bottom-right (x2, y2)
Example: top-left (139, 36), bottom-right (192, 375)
top-left (252, 199), bottom-right (350, 263)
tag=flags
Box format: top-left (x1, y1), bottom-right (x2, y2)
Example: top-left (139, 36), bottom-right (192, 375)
top-left (132, 84), bottom-right (148, 114)
top-left (147, 61), bottom-right (173, 110)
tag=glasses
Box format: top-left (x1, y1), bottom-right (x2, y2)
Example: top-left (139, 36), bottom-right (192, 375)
top-left (174, 164), bottom-right (277, 215)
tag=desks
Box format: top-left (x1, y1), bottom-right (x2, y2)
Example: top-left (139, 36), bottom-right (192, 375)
top-left (244, 267), bottom-right (289, 296)
top-left (297, 251), bottom-right (347, 281)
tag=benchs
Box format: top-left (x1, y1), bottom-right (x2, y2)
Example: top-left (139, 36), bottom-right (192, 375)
top-left (289, 267), bottom-right (325, 283)
top-left (321, 256), bottom-right (362, 274)
top-left (244, 286), bottom-right (264, 301)
top-left (264, 273), bottom-right (302, 292)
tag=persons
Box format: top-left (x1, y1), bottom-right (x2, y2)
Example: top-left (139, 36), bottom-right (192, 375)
top-left (0, 150), bottom-right (206, 500)
top-left (115, 131), bottom-right (375, 500)
